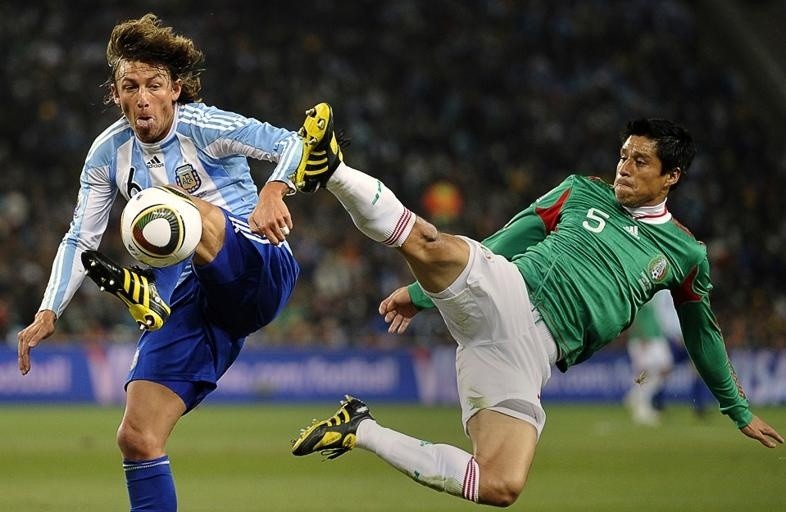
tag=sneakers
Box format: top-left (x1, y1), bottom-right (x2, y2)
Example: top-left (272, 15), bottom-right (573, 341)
top-left (291, 390), bottom-right (374, 463)
top-left (288, 100), bottom-right (345, 195)
top-left (79, 249), bottom-right (171, 332)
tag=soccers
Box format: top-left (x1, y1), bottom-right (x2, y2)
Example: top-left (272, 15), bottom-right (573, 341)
top-left (120, 187), bottom-right (203, 269)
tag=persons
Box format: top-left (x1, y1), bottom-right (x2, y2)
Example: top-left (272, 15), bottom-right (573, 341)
top-left (15, 12), bottom-right (306, 511)
top-left (651, 290), bottom-right (716, 420)
top-left (287, 98), bottom-right (786, 507)
top-left (622, 301), bottom-right (675, 427)
